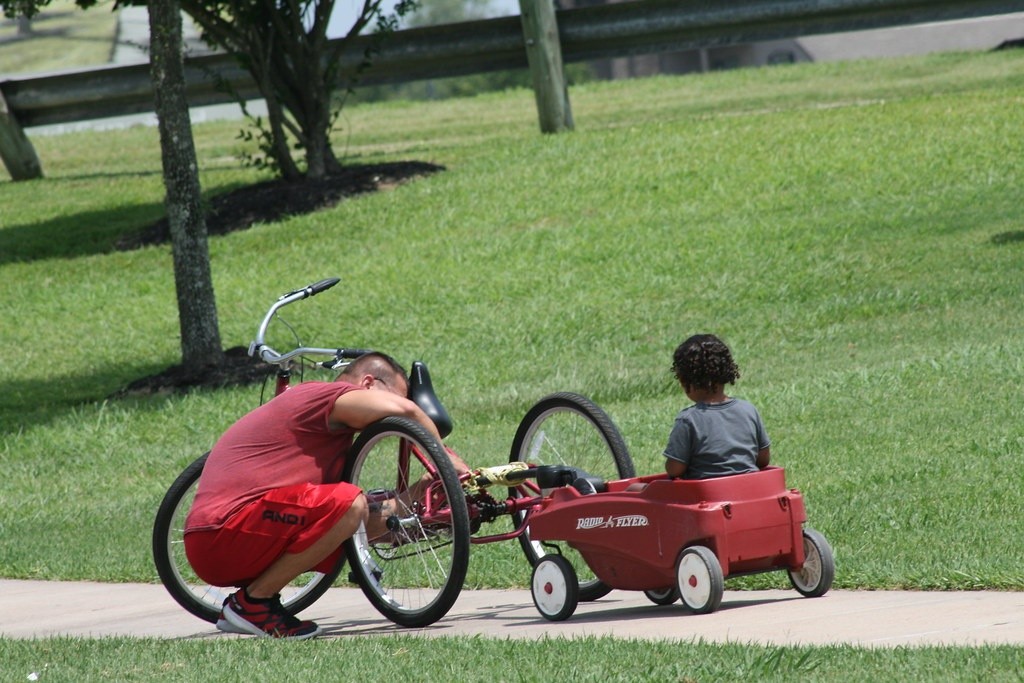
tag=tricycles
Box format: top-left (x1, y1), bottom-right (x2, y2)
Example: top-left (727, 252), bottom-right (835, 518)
top-left (152, 276), bottom-right (639, 628)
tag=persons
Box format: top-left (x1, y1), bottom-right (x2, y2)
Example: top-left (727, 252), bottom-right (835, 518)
top-left (663, 334), bottom-right (770, 480)
top-left (183, 351), bottom-right (471, 642)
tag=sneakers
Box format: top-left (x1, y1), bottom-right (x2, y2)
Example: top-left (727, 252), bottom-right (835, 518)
top-left (217, 607), bottom-right (254, 635)
top-left (223, 585), bottom-right (322, 641)
